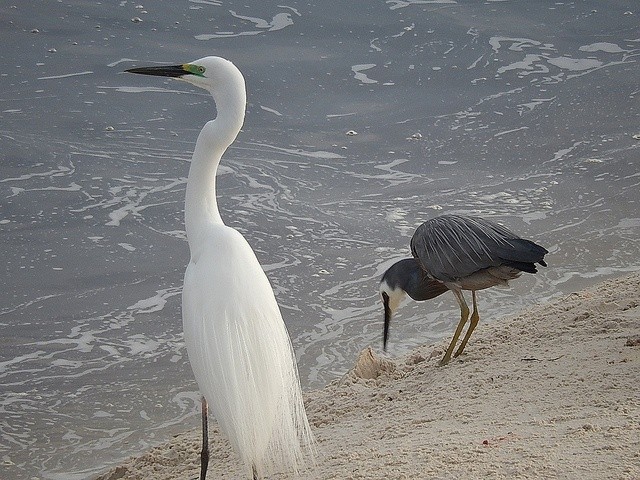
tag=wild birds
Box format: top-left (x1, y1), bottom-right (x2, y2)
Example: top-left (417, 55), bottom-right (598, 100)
top-left (380, 214), bottom-right (548, 368)
top-left (123, 56), bottom-right (326, 480)
